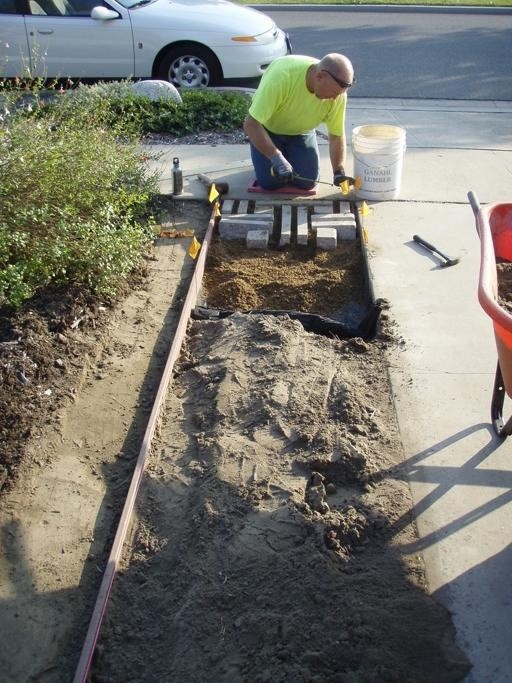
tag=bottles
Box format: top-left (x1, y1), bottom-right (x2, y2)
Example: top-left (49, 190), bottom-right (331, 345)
top-left (171, 157), bottom-right (183, 195)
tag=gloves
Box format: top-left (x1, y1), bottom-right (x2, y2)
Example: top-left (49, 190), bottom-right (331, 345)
top-left (333, 169), bottom-right (355, 187)
top-left (271, 153), bottom-right (297, 184)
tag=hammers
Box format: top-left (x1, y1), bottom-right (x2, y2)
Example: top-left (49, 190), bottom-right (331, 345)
top-left (413, 235), bottom-right (460, 267)
top-left (197, 173), bottom-right (229, 195)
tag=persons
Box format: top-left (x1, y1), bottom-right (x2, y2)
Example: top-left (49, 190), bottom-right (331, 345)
top-left (243, 51), bottom-right (356, 192)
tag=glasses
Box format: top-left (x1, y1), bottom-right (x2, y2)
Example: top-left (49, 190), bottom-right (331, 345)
top-left (321, 69), bottom-right (357, 88)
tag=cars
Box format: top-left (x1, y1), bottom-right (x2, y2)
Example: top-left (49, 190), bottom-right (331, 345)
top-left (0, 1), bottom-right (294, 87)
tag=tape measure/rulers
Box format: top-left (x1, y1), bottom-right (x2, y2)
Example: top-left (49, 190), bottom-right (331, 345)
top-left (270, 164), bottom-right (333, 186)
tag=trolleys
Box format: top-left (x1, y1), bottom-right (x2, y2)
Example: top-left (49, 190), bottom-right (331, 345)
top-left (468, 186), bottom-right (511, 433)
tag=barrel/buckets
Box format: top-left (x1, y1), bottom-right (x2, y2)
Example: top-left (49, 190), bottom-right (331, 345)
top-left (351, 125), bottom-right (407, 200)
